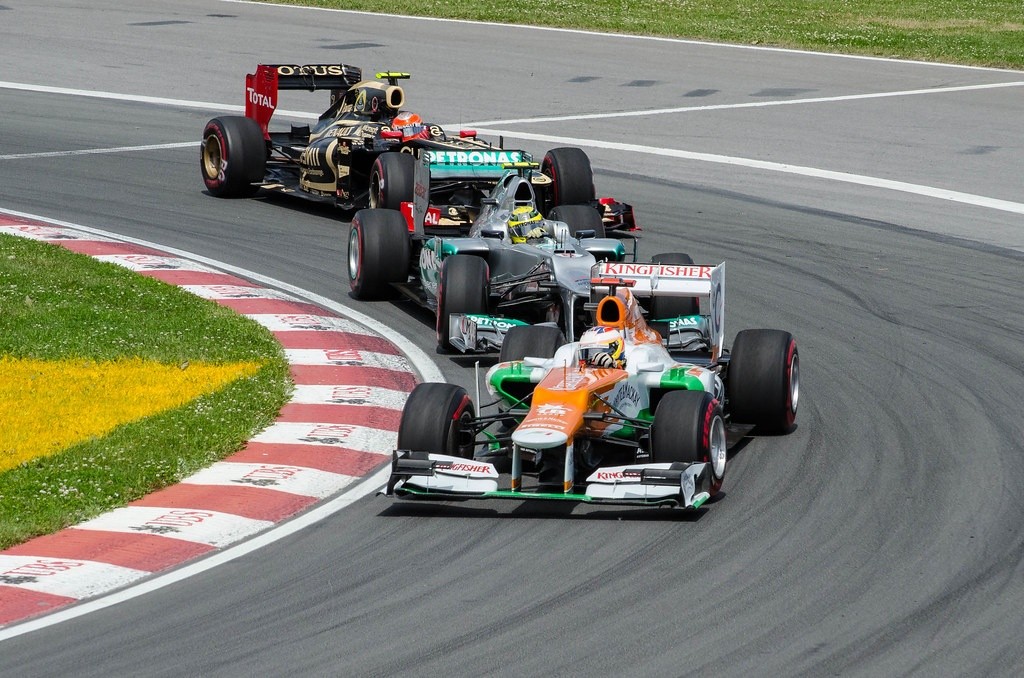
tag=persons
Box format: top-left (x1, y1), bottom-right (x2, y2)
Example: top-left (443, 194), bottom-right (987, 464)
top-left (390, 111), bottom-right (429, 143)
top-left (576, 326), bottom-right (628, 373)
top-left (508, 206), bottom-right (558, 247)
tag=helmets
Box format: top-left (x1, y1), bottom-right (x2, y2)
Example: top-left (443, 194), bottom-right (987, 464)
top-left (508, 206), bottom-right (546, 244)
top-left (578, 325), bottom-right (626, 368)
top-left (392, 112), bottom-right (424, 143)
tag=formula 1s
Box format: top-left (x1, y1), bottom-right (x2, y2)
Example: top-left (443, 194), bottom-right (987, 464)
top-left (347, 146), bottom-right (712, 358)
top-left (376, 259), bottom-right (802, 513)
top-left (200, 64), bottom-right (642, 232)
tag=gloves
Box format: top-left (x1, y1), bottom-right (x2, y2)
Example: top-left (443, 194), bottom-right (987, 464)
top-left (591, 352), bottom-right (618, 368)
top-left (526, 227), bottom-right (547, 239)
